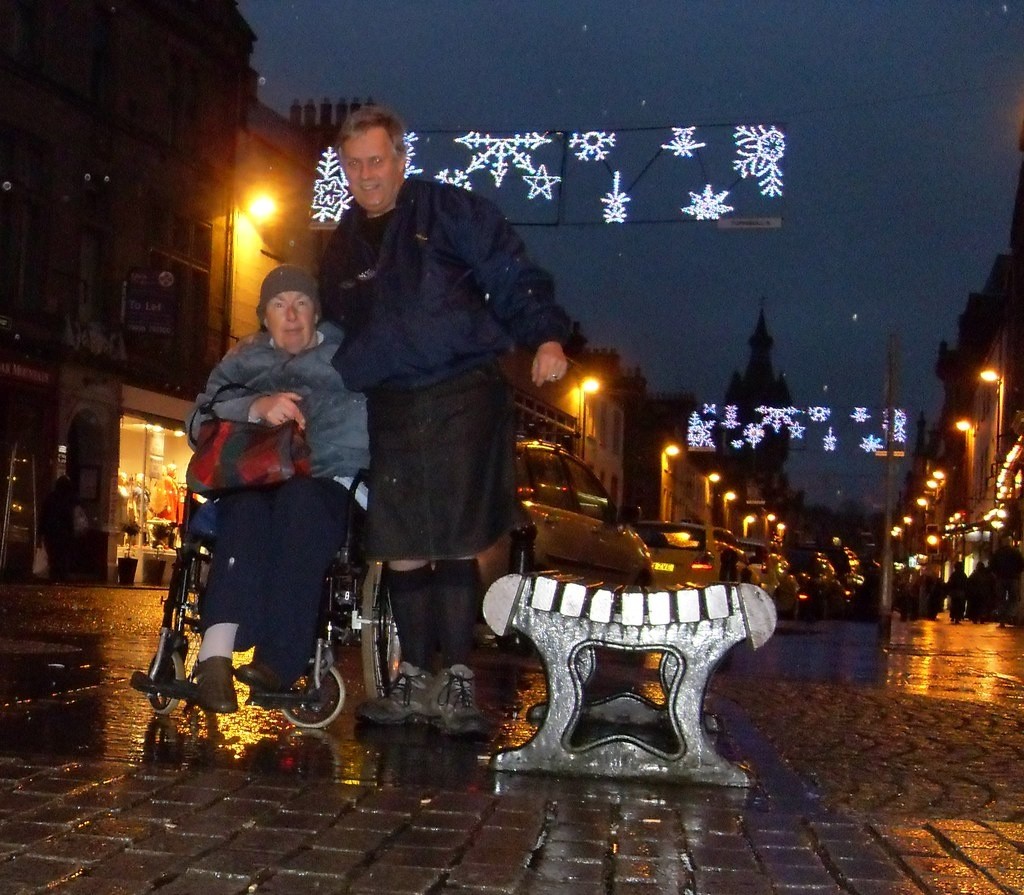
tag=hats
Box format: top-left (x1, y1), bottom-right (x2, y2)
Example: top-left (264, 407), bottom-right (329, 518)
top-left (255, 265), bottom-right (325, 326)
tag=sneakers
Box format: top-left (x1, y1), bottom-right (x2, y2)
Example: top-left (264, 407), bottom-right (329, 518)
top-left (352, 670), bottom-right (442, 728)
top-left (430, 671), bottom-right (489, 737)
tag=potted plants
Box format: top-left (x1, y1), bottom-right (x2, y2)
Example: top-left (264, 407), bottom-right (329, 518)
top-left (118, 520), bottom-right (140, 586)
top-left (142, 522), bottom-right (170, 586)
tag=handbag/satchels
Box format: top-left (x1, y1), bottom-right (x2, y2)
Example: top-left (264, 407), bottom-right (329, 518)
top-left (185, 383), bottom-right (309, 493)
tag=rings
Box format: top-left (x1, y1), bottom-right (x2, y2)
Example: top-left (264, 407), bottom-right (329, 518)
top-left (553, 374), bottom-right (558, 378)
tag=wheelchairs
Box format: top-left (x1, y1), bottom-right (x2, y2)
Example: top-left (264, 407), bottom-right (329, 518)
top-left (127, 458), bottom-right (399, 734)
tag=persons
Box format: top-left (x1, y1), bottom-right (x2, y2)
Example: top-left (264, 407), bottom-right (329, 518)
top-left (38, 473), bottom-right (79, 582)
top-left (114, 472), bottom-right (131, 544)
top-left (987, 530), bottom-right (1024, 629)
top-left (911, 566), bottom-right (947, 621)
top-left (740, 566), bottom-right (754, 584)
top-left (968, 562), bottom-right (996, 624)
top-left (133, 472), bottom-right (150, 529)
top-left (164, 464), bottom-right (180, 551)
top-left (720, 544), bottom-right (737, 584)
top-left (180, 261), bottom-right (371, 713)
top-left (312, 100), bottom-right (573, 734)
top-left (946, 561), bottom-right (969, 623)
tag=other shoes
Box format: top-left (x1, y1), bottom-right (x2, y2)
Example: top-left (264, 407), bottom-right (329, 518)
top-left (192, 655), bottom-right (239, 713)
top-left (235, 659), bottom-right (281, 691)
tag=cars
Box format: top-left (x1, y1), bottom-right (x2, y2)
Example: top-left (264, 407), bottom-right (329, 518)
top-left (510, 438), bottom-right (656, 590)
top-left (636, 519), bottom-right (875, 624)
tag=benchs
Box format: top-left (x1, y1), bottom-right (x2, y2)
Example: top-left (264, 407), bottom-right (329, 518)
top-left (481, 570), bottom-right (777, 788)
